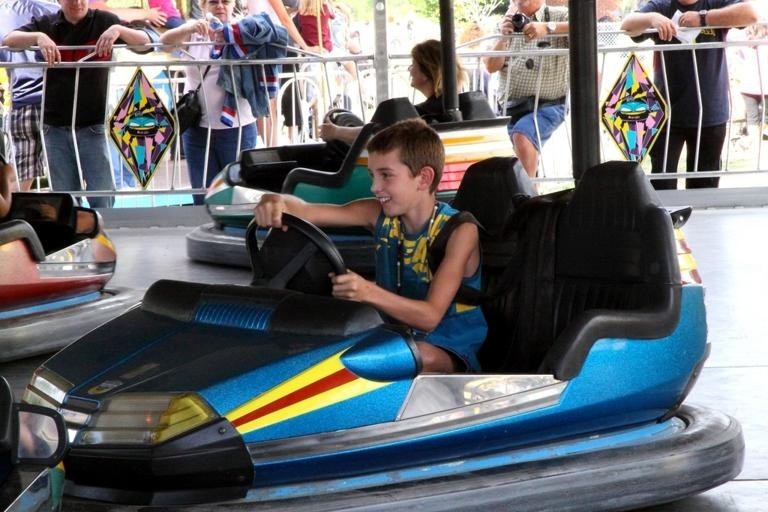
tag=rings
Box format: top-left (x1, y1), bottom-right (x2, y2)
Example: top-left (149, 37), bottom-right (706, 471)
top-left (528, 33), bottom-right (532, 38)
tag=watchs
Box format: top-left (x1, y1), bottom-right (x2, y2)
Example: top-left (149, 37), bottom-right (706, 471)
top-left (699, 9), bottom-right (708, 28)
top-left (547, 20), bottom-right (557, 35)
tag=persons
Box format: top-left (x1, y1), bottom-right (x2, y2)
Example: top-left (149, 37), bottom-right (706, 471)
top-left (620, 0), bottom-right (757, 190)
top-left (255, 117), bottom-right (490, 376)
top-left (0, 163), bottom-right (16, 217)
top-left (483, 0), bottom-right (571, 179)
top-left (317, 40), bottom-right (465, 150)
top-left (736, 16), bottom-right (768, 148)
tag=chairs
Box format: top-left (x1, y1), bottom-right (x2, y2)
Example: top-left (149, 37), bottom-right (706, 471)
top-left (370, 97), bottom-right (422, 128)
top-left (455, 156), bottom-right (528, 375)
top-left (537, 163), bottom-right (682, 380)
top-left (458, 90), bottom-right (496, 119)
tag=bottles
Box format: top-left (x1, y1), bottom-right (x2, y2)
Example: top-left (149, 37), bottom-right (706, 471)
top-left (206, 13), bottom-right (223, 32)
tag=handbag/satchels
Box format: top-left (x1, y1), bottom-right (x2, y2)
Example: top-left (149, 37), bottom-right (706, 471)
top-left (497, 94), bottom-right (539, 127)
top-left (170, 91), bottom-right (202, 135)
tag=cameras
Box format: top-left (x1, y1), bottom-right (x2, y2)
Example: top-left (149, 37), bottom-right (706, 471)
top-left (511, 12), bottom-right (531, 32)
